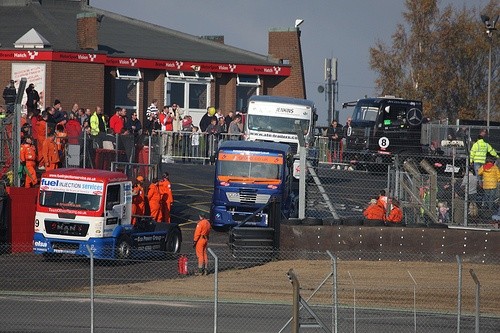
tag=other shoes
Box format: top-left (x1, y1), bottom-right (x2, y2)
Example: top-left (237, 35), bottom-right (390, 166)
top-left (331, 164), bottom-right (336, 169)
top-left (204, 268), bottom-right (208, 275)
top-left (337, 165), bottom-right (341, 169)
top-left (195, 268), bottom-right (203, 276)
top-left (344, 166), bottom-right (348, 170)
top-left (348, 166), bottom-right (354, 170)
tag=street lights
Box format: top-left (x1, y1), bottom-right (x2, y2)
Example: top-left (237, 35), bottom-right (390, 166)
top-left (480, 13), bottom-right (499, 128)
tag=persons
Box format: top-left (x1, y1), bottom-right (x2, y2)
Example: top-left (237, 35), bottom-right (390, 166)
top-left (191, 213), bottom-right (212, 275)
top-left (362, 199), bottom-right (385, 221)
top-left (342, 116), bottom-right (357, 171)
top-left (0, 79), bottom-right (242, 188)
top-left (419, 183), bottom-right (451, 223)
top-left (378, 190), bottom-right (390, 212)
top-left (470, 135), bottom-right (499, 179)
top-left (477, 156), bottom-right (500, 209)
top-left (326, 120), bottom-right (344, 169)
top-left (131, 172), bottom-right (173, 223)
top-left (386, 200), bottom-right (403, 224)
top-left (433, 127), bottom-right (472, 158)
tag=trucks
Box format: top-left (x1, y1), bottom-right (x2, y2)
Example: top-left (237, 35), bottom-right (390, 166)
top-left (244, 96), bottom-right (321, 182)
top-left (31, 167), bottom-right (182, 267)
top-left (209, 139), bottom-right (301, 233)
top-left (344, 95), bottom-right (500, 174)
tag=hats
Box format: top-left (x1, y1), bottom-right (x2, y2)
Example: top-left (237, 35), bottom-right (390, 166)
top-left (7, 80), bottom-right (16, 88)
top-left (199, 211), bottom-right (206, 216)
top-left (23, 123), bottom-right (32, 128)
top-left (137, 176), bottom-right (145, 181)
top-left (26, 135), bottom-right (34, 139)
top-left (477, 135), bottom-right (484, 139)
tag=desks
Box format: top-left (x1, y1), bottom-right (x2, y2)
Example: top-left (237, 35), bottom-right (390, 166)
top-left (95, 148), bottom-right (128, 173)
